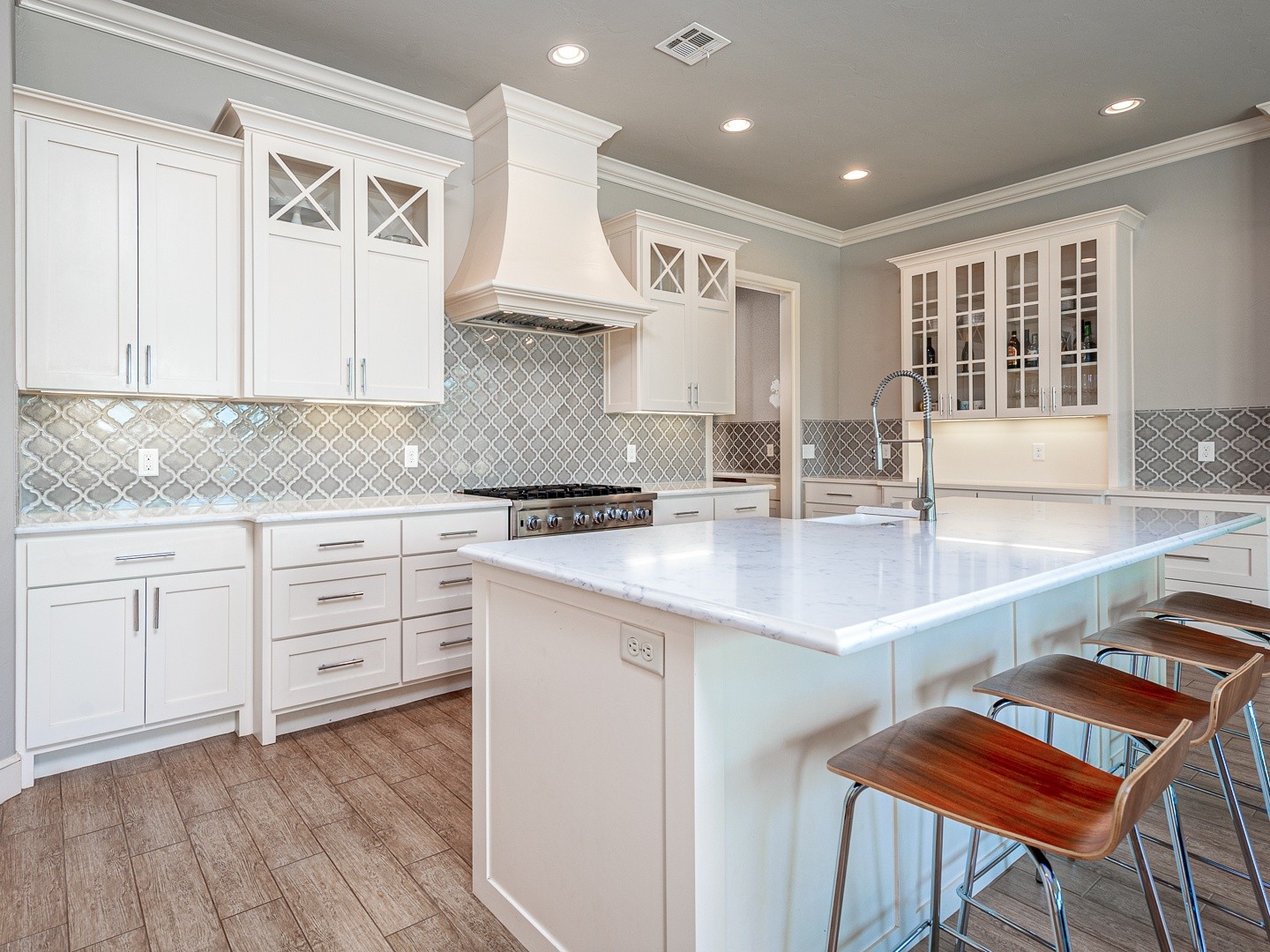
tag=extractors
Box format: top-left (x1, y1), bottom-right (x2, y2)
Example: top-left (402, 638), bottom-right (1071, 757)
top-left (444, 83), bottom-right (659, 339)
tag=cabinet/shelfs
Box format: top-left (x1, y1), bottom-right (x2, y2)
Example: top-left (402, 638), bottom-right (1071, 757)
top-left (207, 96), bottom-right (466, 411)
top-left (995, 203), bottom-right (1148, 415)
top-left (803, 475), bottom-right (881, 520)
top-left (1104, 488), bottom-right (1270, 607)
top-left (240, 497), bottom-right (518, 747)
top-left (620, 480), bottom-right (778, 528)
top-left (884, 241), bottom-right (994, 423)
top-left (11, 79), bottom-right (244, 404)
top-left (602, 208), bottom-right (753, 418)
top-left (11, 506), bottom-right (255, 792)
top-left (712, 469), bottom-right (780, 518)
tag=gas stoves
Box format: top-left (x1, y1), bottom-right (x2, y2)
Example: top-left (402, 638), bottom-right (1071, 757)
top-left (452, 483), bottom-right (658, 537)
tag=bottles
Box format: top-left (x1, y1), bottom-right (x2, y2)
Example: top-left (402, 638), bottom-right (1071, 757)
top-left (1073, 320), bottom-right (1084, 363)
top-left (926, 337), bottom-right (936, 376)
top-left (1081, 320), bottom-right (1097, 363)
top-left (961, 337), bottom-right (974, 373)
top-left (1007, 328), bottom-right (1039, 369)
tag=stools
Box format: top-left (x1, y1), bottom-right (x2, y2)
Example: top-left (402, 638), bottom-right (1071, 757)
top-left (1135, 590), bottom-right (1270, 699)
top-left (971, 651), bottom-right (1270, 952)
top-left (816, 701), bottom-right (1196, 952)
top-left (1079, 614), bottom-right (1270, 821)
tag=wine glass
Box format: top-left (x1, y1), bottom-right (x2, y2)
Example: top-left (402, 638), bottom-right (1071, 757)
top-left (1062, 373), bottom-right (1097, 406)
top-left (1010, 377), bottom-right (1038, 408)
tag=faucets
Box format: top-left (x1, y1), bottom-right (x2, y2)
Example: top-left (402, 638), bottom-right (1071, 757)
top-left (869, 369), bottom-right (938, 522)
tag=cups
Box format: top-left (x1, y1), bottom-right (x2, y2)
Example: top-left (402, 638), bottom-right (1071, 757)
top-left (959, 401), bottom-right (969, 410)
top-left (973, 400), bottom-right (985, 410)
top-left (930, 403), bottom-right (938, 411)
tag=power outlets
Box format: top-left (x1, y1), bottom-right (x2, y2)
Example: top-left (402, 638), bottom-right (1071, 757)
top-left (882, 443), bottom-right (892, 459)
top-left (1032, 442), bottom-right (1045, 461)
top-left (137, 446), bottom-right (160, 478)
top-left (766, 444), bottom-right (774, 458)
top-left (1196, 440), bottom-right (1216, 463)
top-left (404, 444), bottom-right (420, 469)
top-left (618, 620), bottom-right (668, 678)
top-left (1196, 510), bottom-right (1217, 529)
top-left (627, 444), bottom-right (637, 463)
